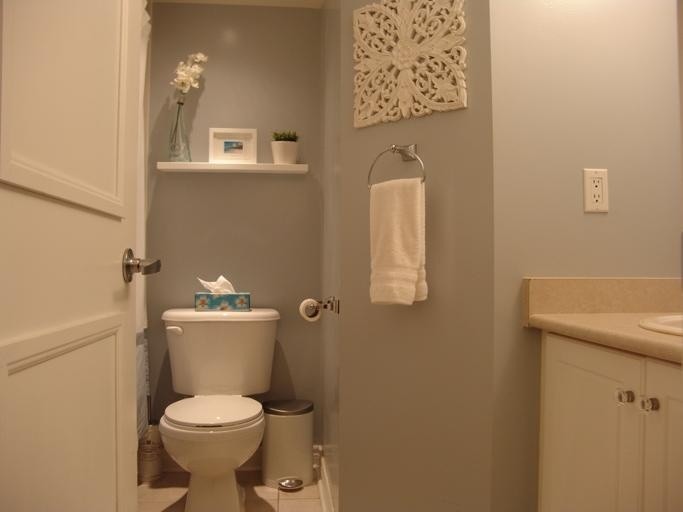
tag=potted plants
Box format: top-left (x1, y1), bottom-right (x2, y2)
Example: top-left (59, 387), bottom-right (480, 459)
top-left (270, 131), bottom-right (299, 166)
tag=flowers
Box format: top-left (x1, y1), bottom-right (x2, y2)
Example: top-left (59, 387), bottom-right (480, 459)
top-left (170, 50), bottom-right (208, 150)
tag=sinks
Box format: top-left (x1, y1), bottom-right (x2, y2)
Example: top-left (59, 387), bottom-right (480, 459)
top-left (640, 312), bottom-right (683, 336)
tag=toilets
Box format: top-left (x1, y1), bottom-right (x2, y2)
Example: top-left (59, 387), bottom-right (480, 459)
top-left (159, 309), bottom-right (280, 512)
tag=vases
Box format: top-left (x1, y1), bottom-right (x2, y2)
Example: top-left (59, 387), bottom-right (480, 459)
top-left (170, 102), bottom-right (190, 162)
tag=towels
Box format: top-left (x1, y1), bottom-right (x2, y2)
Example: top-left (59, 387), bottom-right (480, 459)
top-left (367, 176), bottom-right (429, 306)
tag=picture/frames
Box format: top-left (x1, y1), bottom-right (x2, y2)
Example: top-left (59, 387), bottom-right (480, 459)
top-left (207, 127), bottom-right (258, 165)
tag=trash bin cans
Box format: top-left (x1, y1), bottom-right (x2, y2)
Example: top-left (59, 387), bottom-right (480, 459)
top-left (261, 400), bottom-right (315, 490)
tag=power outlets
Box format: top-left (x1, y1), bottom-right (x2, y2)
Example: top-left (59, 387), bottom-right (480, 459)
top-left (584, 169), bottom-right (610, 213)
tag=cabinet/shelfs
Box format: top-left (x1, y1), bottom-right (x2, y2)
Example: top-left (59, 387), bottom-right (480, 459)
top-left (539, 336), bottom-right (682, 512)
